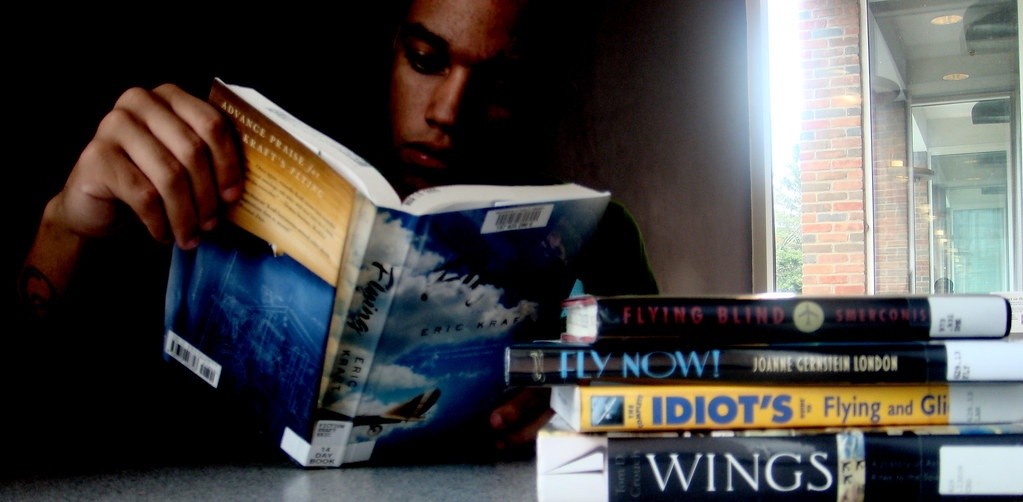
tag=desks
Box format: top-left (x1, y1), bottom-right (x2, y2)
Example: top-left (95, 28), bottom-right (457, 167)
top-left (0, 461), bottom-right (539, 502)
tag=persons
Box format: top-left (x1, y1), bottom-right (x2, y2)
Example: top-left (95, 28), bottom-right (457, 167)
top-left (933, 277), bottom-right (954, 295)
top-left (0, 0), bottom-right (659, 464)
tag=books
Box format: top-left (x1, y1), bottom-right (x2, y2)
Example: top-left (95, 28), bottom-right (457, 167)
top-left (501, 293), bottom-right (1023, 502)
top-left (162, 77), bottom-right (611, 467)
